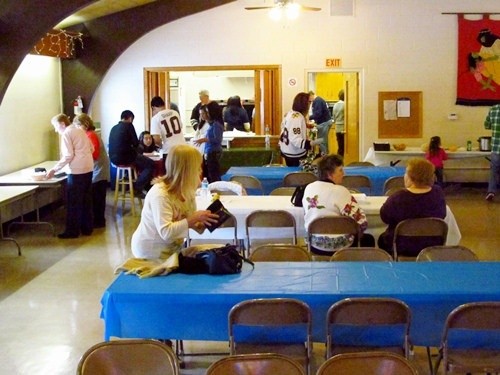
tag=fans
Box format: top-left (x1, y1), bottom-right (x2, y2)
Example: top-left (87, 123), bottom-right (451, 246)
top-left (245, 0), bottom-right (321, 12)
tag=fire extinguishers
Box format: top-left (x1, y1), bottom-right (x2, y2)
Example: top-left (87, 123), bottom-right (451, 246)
top-left (74, 95), bottom-right (82, 116)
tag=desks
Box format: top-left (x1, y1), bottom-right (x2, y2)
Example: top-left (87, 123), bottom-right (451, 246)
top-left (99, 261), bottom-right (500, 375)
top-left (221, 166), bottom-right (406, 197)
top-left (182, 194), bottom-right (461, 260)
top-left (0, 160), bottom-right (68, 256)
top-left (363, 146), bottom-right (492, 183)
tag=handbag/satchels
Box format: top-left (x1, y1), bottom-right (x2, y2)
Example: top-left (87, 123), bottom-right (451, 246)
top-left (178, 242), bottom-right (254, 276)
top-left (291, 183), bottom-right (308, 207)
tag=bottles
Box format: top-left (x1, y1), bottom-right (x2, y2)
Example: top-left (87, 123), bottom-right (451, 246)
top-left (265, 124), bottom-right (270, 135)
top-left (466, 141), bottom-right (471, 151)
top-left (201, 178), bottom-right (210, 201)
top-left (265, 135), bottom-right (270, 149)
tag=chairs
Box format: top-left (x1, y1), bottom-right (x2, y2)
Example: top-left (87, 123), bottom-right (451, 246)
top-left (77, 161), bottom-right (500, 375)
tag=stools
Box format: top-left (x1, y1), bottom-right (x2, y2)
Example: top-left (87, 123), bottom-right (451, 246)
top-left (112, 163), bottom-right (143, 217)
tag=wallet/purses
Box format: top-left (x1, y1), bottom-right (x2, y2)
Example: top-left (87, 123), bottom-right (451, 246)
top-left (205, 199), bottom-right (231, 233)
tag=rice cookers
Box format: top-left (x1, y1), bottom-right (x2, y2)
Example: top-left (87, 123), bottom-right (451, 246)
top-left (477, 137), bottom-right (491, 151)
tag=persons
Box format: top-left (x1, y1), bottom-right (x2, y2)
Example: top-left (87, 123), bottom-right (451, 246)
top-left (377, 157), bottom-right (446, 257)
top-left (484, 103), bottom-right (500, 200)
top-left (189, 90), bottom-right (224, 184)
top-left (72, 113), bottom-right (110, 229)
top-left (109, 110), bottom-right (157, 199)
top-left (223, 96), bottom-right (250, 132)
top-left (426, 135), bottom-right (448, 193)
top-left (130, 145), bottom-right (220, 261)
top-left (302, 153), bottom-right (375, 257)
top-left (45, 114), bottom-right (95, 239)
top-left (278, 92), bottom-right (322, 167)
top-left (308, 90), bottom-right (333, 156)
top-left (332, 89), bottom-right (346, 157)
top-left (150, 96), bottom-right (187, 175)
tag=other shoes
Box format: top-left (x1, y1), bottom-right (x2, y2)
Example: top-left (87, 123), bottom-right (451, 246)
top-left (486, 192), bottom-right (494, 200)
top-left (57, 232), bottom-right (79, 239)
top-left (134, 191), bottom-right (145, 199)
